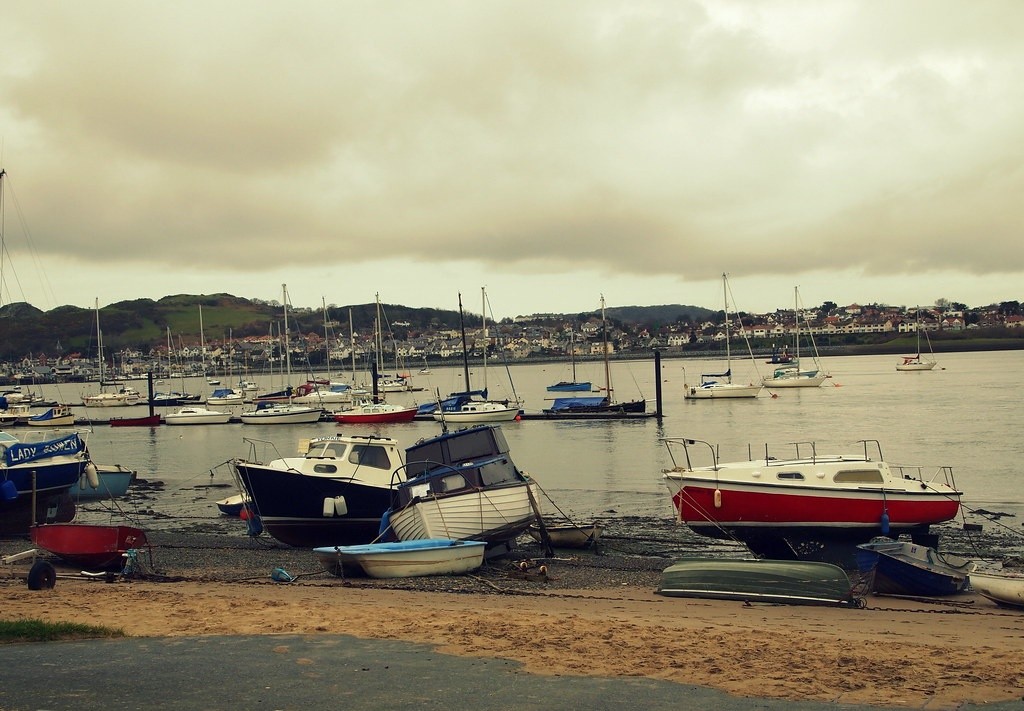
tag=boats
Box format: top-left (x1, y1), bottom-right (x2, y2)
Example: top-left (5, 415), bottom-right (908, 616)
top-left (383, 386), bottom-right (566, 550)
top-left (653, 556), bottom-right (860, 608)
top-left (28, 520), bottom-right (148, 572)
top-left (0, 426), bottom-right (94, 536)
top-left (312, 540), bottom-right (411, 579)
top-left (0, 372), bottom-right (76, 427)
top-left (57, 463), bottom-right (140, 500)
top-left (966, 567), bottom-right (1024, 608)
top-left (857, 540), bottom-right (980, 597)
top-left (338, 536), bottom-right (489, 578)
top-left (658, 435), bottom-right (964, 532)
top-left (225, 433), bottom-right (407, 549)
top-left (765, 333), bottom-right (799, 366)
top-left (968, 571), bottom-right (1024, 609)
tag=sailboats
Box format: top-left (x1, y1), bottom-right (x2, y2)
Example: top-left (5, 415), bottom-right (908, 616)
top-left (147, 325), bottom-right (203, 408)
top-left (92, 327), bottom-right (208, 387)
top-left (203, 292), bottom-right (426, 411)
top-left (432, 285), bottom-right (526, 423)
top-left (240, 282), bottom-right (324, 424)
top-left (684, 271), bottom-right (766, 399)
top-left (546, 340), bottom-right (591, 392)
top-left (761, 286), bottom-right (835, 388)
top-left (332, 291), bottom-right (420, 424)
top-left (206, 327), bottom-right (249, 406)
top-left (894, 304), bottom-right (939, 370)
top-left (161, 303), bottom-right (234, 426)
top-left (408, 291), bottom-right (488, 421)
top-left (541, 292), bottom-right (647, 414)
top-left (79, 296), bottom-right (144, 408)
